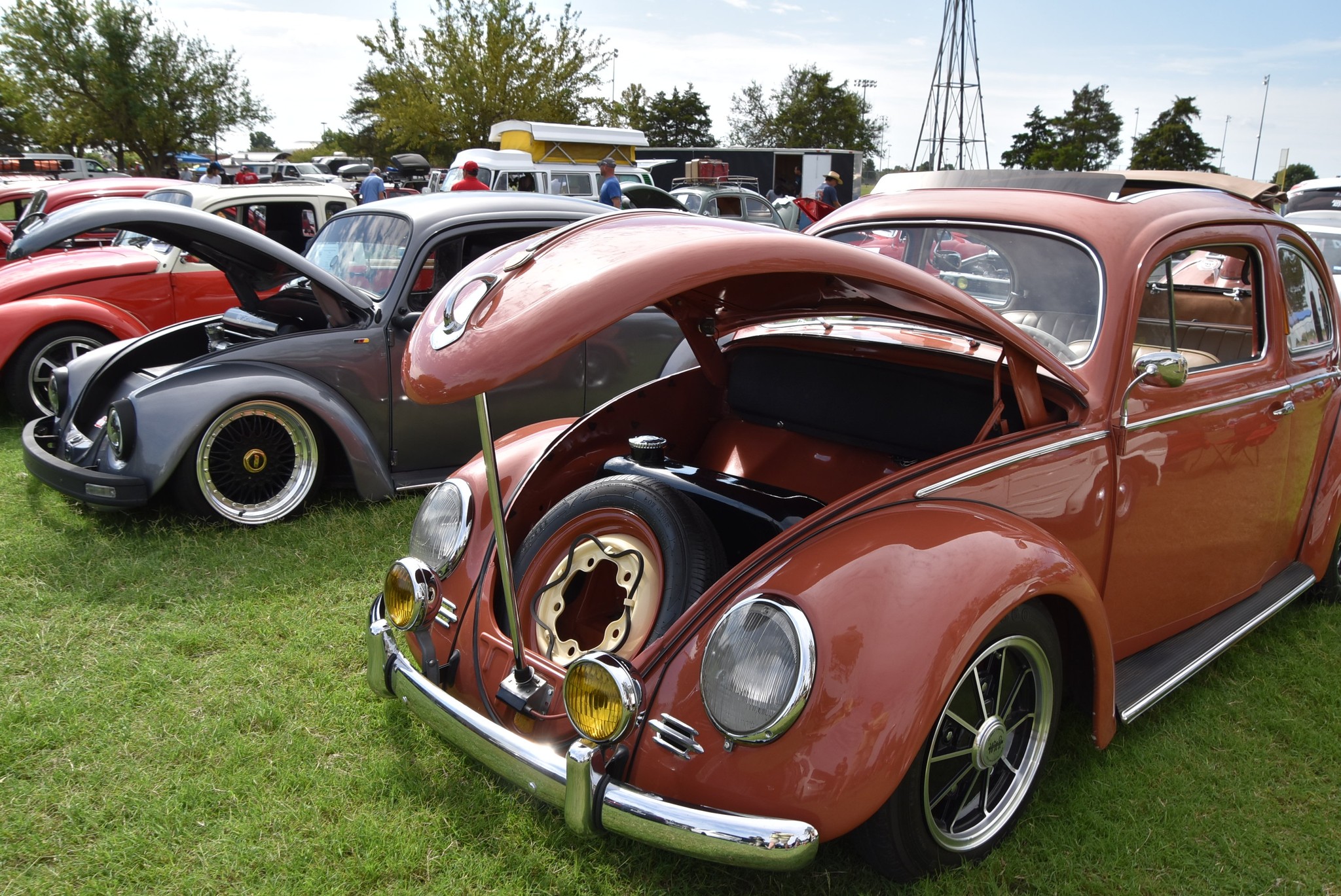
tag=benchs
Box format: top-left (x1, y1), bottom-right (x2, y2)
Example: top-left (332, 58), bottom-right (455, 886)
top-left (1002, 310), bottom-right (1253, 361)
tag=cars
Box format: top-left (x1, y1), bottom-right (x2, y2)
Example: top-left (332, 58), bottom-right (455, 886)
top-left (1158, 210), bottom-right (1341, 300)
top-left (619, 180), bottom-right (800, 231)
top-left (0, 145), bottom-right (448, 209)
top-left (0, 188), bottom-right (737, 529)
top-left (0, 177), bottom-right (197, 269)
top-left (1280, 178), bottom-right (1341, 218)
top-left (367, 167), bottom-right (1341, 873)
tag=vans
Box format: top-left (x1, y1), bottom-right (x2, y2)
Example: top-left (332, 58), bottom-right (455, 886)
top-left (423, 148), bottom-right (655, 228)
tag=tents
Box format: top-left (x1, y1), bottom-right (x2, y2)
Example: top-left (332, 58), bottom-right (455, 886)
top-left (153, 151), bottom-right (211, 163)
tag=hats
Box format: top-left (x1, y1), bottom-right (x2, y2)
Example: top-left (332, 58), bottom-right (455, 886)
top-left (458, 161), bottom-right (479, 172)
top-left (822, 171), bottom-right (843, 185)
top-left (597, 158), bottom-right (616, 168)
top-left (209, 161), bottom-right (224, 171)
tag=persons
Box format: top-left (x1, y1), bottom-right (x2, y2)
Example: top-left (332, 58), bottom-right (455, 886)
top-left (198, 161), bottom-right (224, 185)
top-left (166, 164), bottom-right (181, 179)
top-left (551, 176), bottom-right (563, 194)
top-left (596, 158), bottom-right (622, 210)
top-left (450, 161), bottom-right (490, 190)
top-left (270, 172), bottom-right (285, 183)
top-left (235, 166), bottom-right (248, 185)
top-left (180, 166), bottom-right (194, 182)
top-left (359, 167), bottom-right (385, 205)
top-left (766, 185), bottom-right (783, 203)
top-left (815, 171), bottom-right (843, 208)
top-left (794, 165), bottom-right (802, 198)
top-left (241, 168), bottom-right (260, 184)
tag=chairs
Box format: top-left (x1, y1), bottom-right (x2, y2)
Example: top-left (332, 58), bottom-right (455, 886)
top-left (1056, 339), bottom-right (1220, 369)
